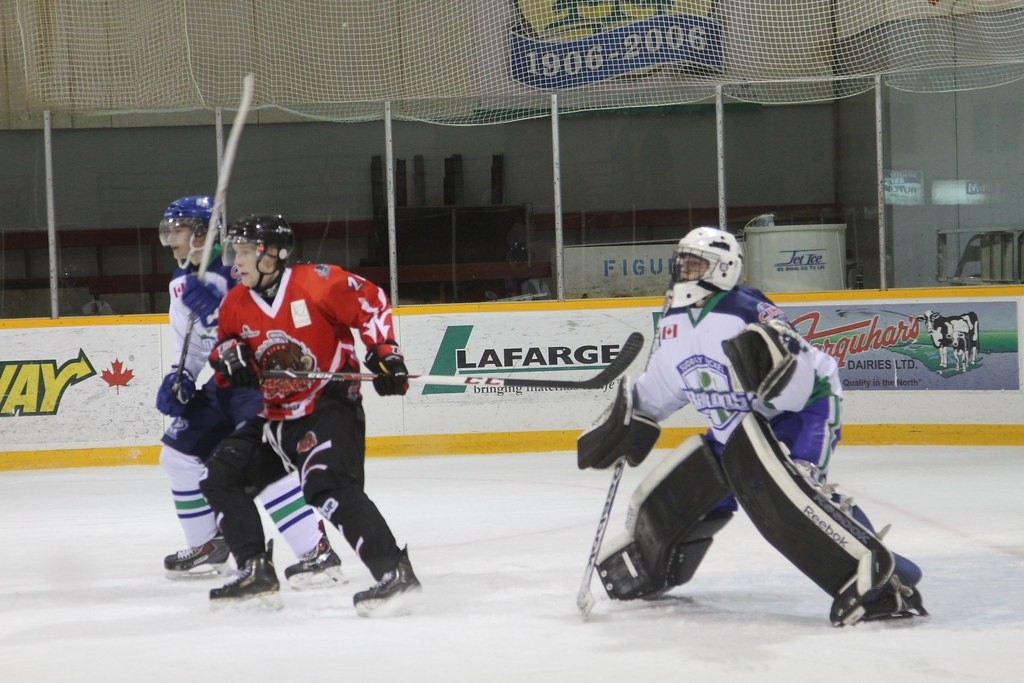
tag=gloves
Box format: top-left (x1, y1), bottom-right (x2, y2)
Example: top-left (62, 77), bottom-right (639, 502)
top-left (365, 346), bottom-right (412, 395)
top-left (155, 367), bottom-right (197, 415)
top-left (209, 333), bottom-right (262, 384)
top-left (182, 278), bottom-right (224, 326)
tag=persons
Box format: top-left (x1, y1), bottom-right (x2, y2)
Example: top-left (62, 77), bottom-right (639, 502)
top-left (153, 196), bottom-right (423, 616)
top-left (573, 226), bottom-right (932, 631)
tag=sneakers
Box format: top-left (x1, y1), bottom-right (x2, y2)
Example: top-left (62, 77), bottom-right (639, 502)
top-left (354, 544), bottom-right (425, 616)
top-left (206, 539), bottom-right (281, 609)
top-left (283, 518), bottom-right (349, 590)
top-left (162, 531), bottom-right (232, 576)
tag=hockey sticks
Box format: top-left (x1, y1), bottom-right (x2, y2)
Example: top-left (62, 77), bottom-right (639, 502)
top-left (172, 75), bottom-right (255, 393)
top-left (258, 330), bottom-right (645, 390)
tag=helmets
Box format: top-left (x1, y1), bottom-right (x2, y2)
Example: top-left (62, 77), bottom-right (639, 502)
top-left (226, 211), bottom-right (290, 262)
top-left (669, 227), bottom-right (742, 308)
top-left (163, 196), bottom-right (221, 239)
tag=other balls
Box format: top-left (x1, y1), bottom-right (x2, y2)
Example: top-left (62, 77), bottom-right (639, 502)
top-left (577, 456), bottom-right (630, 621)
